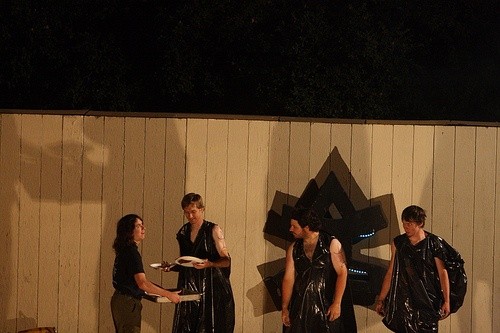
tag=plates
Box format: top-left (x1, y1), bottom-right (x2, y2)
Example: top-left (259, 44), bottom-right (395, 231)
top-left (150, 262), bottom-right (175, 270)
top-left (176, 256), bottom-right (203, 267)
top-left (145, 290), bottom-right (181, 296)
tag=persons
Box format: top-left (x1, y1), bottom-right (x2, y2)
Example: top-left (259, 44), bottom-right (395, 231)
top-left (110, 213), bottom-right (184, 333)
top-left (375, 205), bottom-right (468, 333)
top-left (282, 207), bottom-right (357, 333)
top-left (172, 192), bottom-right (236, 333)
